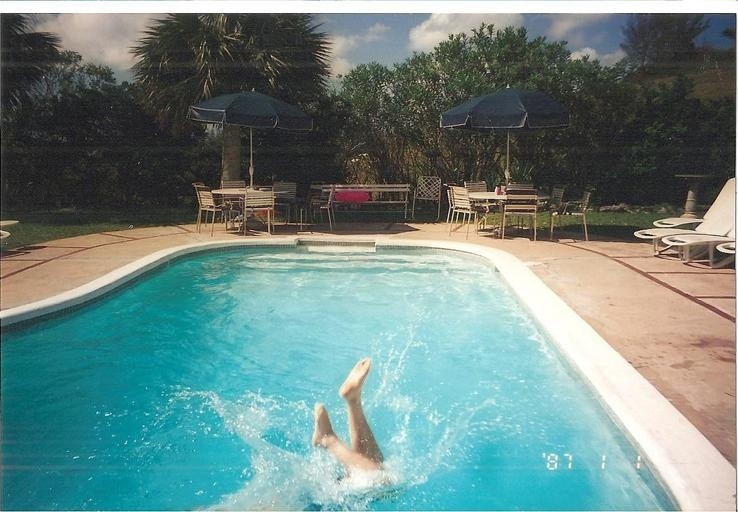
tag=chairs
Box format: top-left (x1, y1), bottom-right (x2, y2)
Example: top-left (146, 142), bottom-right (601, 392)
top-left (191, 179), bottom-right (338, 236)
top-left (633, 177), bottom-right (736, 268)
top-left (411, 175), bottom-right (441, 222)
top-left (444, 180), bottom-right (592, 242)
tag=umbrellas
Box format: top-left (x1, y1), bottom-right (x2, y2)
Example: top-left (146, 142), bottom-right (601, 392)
top-left (186, 88), bottom-right (315, 190)
top-left (439, 84), bottom-right (570, 184)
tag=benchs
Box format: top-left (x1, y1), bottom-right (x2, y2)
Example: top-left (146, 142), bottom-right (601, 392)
top-left (311, 183), bottom-right (415, 218)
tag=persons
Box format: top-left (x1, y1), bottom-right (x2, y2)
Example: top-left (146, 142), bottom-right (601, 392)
top-left (312, 357), bottom-right (383, 478)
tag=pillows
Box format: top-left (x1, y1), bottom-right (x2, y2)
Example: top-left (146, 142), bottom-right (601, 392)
top-left (335, 192), bottom-right (368, 203)
